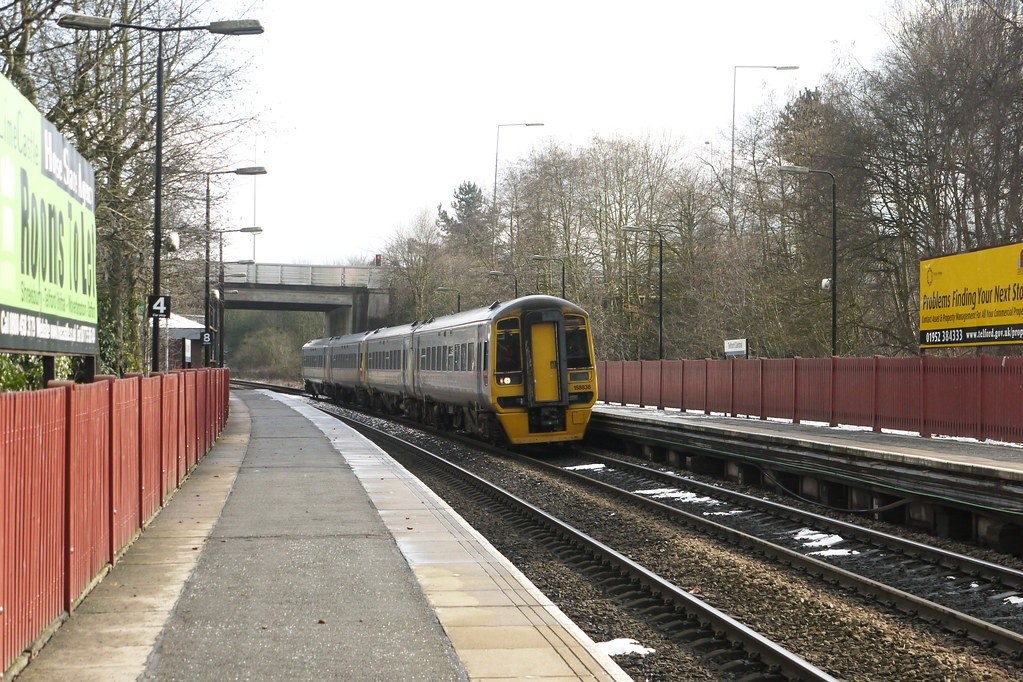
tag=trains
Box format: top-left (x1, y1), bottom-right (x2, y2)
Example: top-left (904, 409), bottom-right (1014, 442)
top-left (299, 294), bottom-right (599, 450)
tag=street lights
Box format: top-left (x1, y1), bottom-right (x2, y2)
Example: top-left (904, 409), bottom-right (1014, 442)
top-left (776, 165), bottom-right (837, 358)
top-left (438, 287), bottom-right (461, 314)
top-left (493, 122), bottom-right (545, 208)
top-left (490, 270), bottom-right (518, 300)
top-left (729, 64), bottom-right (799, 243)
top-left (622, 225), bottom-right (663, 359)
top-left (56, 13), bottom-right (268, 375)
top-left (534, 255), bottom-right (565, 300)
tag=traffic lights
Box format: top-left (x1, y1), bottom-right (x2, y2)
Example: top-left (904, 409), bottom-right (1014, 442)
top-left (376, 254), bottom-right (381, 266)
